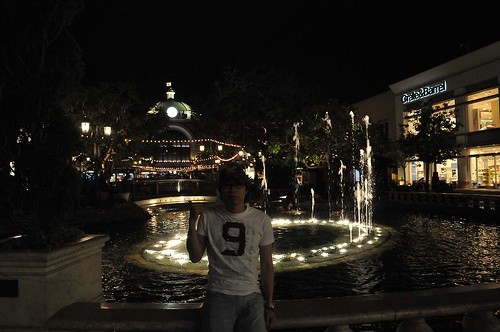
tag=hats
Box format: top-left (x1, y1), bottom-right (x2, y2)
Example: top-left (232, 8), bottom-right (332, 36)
top-left (219, 166), bottom-right (243, 179)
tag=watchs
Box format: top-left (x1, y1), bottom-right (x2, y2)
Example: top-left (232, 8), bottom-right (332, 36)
top-left (265, 303), bottom-right (276, 311)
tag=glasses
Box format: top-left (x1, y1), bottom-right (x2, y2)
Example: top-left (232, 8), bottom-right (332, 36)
top-left (221, 183), bottom-right (244, 189)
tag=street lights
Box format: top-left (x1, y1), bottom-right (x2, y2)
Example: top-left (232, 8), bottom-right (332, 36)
top-left (80, 114), bottom-right (113, 200)
top-left (198, 140), bottom-right (226, 181)
top-left (132, 153), bottom-right (143, 182)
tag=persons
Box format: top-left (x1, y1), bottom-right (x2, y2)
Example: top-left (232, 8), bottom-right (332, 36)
top-left (187, 162), bottom-right (280, 332)
